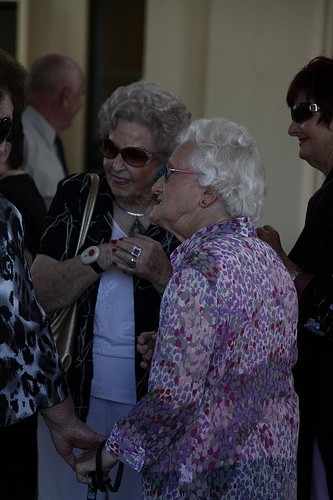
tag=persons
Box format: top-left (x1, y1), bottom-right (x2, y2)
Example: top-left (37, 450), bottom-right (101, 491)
top-left (0, 48), bottom-right (108, 499)
top-left (29, 80), bottom-right (192, 500)
top-left (22, 54), bottom-right (85, 210)
top-left (77, 119), bottom-right (299, 500)
top-left (257, 56), bottom-right (333, 499)
top-left (3, 132), bottom-right (48, 247)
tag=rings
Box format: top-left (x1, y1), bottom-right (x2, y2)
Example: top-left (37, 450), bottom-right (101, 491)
top-left (131, 246), bottom-right (142, 257)
top-left (130, 259), bottom-right (136, 267)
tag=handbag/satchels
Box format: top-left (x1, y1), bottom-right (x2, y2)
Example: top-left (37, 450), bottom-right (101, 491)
top-left (45, 173), bottom-right (99, 371)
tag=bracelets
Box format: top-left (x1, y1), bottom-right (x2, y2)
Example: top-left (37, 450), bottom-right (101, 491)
top-left (80, 246), bottom-right (105, 276)
top-left (290, 268), bottom-right (302, 281)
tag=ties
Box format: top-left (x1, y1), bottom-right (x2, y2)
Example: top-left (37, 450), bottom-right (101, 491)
top-left (54, 134), bottom-right (68, 178)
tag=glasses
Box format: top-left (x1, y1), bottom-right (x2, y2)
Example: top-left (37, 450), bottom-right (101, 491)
top-left (0, 117), bottom-right (10, 144)
top-left (291, 101), bottom-right (322, 125)
top-left (165, 163), bottom-right (205, 180)
top-left (98, 136), bottom-right (171, 167)
